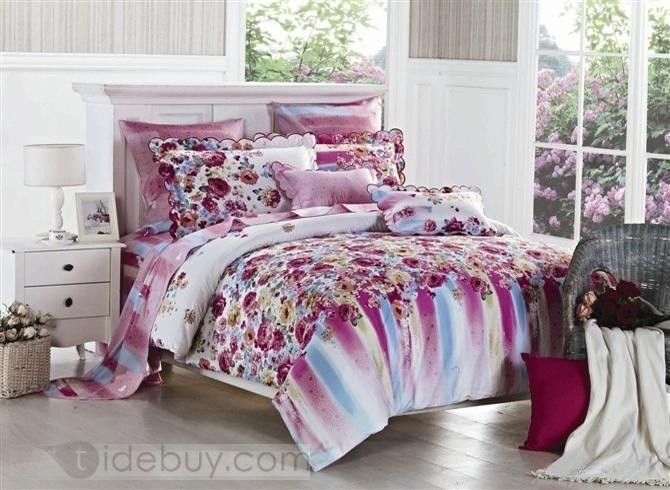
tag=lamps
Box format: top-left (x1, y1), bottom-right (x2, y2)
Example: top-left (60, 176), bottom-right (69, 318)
top-left (23, 144), bottom-right (86, 239)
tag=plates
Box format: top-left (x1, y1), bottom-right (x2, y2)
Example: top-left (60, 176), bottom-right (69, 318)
top-left (41, 239), bottom-right (73, 248)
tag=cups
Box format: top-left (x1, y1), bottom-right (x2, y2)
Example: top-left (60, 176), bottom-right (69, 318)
top-left (49, 230), bottom-right (65, 240)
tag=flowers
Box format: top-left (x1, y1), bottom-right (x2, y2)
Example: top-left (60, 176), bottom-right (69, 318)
top-left (576, 268), bottom-right (641, 331)
top-left (2, 301), bottom-right (54, 344)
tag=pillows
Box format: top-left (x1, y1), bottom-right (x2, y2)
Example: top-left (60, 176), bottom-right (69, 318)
top-left (520, 350), bottom-right (590, 455)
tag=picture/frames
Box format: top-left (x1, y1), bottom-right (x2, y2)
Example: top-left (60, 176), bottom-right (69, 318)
top-left (74, 191), bottom-right (120, 243)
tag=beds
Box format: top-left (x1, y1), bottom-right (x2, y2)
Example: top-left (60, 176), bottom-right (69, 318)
top-left (73, 80), bottom-right (574, 442)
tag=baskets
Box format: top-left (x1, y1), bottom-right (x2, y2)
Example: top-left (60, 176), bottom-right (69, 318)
top-left (0, 335), bottom-right (51, 399)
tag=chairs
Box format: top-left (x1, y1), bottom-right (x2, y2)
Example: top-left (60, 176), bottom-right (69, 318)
top-left (558, 224), bottom-right (670, 386)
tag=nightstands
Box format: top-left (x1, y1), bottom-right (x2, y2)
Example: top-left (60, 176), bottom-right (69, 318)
top-left (2, 238), bottom-right (126, 361)
